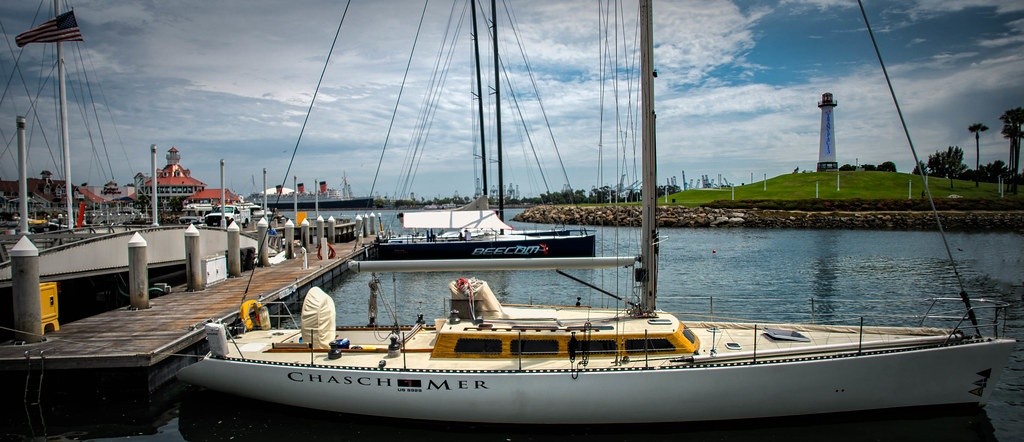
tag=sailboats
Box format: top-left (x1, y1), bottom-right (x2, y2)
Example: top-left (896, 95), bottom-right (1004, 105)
top-left (169, 0), bottom-right (1018, 427)
top-left (360, 0), bottom-right (601, 261)
top-left (0, 0), bottom-right (275, 317)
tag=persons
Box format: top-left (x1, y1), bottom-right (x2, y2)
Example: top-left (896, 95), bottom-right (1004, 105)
top-left (464, 228), bottom-right (468, 232)
top-left (268, 223), bottom-right (278, 247)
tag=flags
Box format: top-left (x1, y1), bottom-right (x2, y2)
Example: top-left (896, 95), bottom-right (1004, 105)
top-left (297, 182), bottom-right (304, 192)
top-left (319, 180), bottom-right (327, 193)
top-left (275, 185), bottom-right (282, 192)
